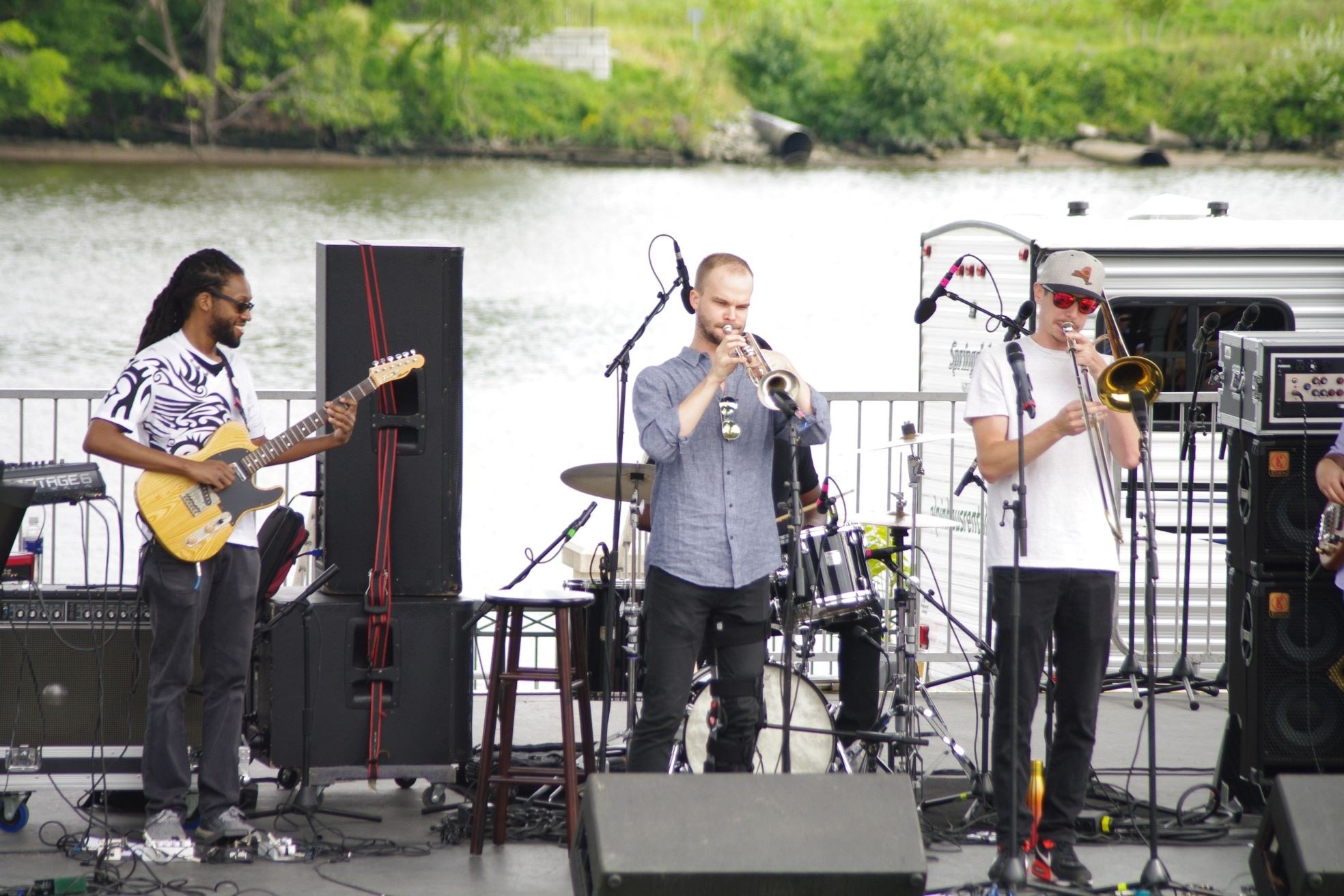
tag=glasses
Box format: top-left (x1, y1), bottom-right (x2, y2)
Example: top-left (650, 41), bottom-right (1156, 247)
top-left (718, 396), bottom-right (743, 443)
top-left (208, 287), bottom-right (255, 314)
top-left (1042, 284), bottom-right (1101, 315)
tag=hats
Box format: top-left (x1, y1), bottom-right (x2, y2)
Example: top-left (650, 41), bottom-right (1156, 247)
top-left (1038, 249), bottom-right (1110, 304)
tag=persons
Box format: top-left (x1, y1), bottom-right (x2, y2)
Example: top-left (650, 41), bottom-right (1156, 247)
top-left (1316, 419), bottom-right (1344, 590)
top-left (962, 247), bottom-right (1140, 887)
top-left (625, 253), bottom-right (831, 775)
top-left (82, 250), bottom-right (356, 841)
top-left (637, 334), bottom-right (883, 763)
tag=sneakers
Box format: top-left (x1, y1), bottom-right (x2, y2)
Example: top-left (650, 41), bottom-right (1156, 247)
top-left (1030, 841), bottom-right (1093, 887)
top-left (195, 806), bottom-right (256, 840)
top-left (144, 810), bottom-right (186, 841)
top-left (996, 839), bottom-right (1033, 877)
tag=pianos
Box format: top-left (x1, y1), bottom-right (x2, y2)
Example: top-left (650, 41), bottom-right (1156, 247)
top-left (0, 459), bottom-right (107, 582)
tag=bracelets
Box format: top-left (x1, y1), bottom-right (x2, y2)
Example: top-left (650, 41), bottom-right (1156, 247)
top-left (802, 524), bottom-right (815, 529)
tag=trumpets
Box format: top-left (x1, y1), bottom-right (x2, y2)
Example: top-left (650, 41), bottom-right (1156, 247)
top-left (722, 325), bottom-right (800, 410)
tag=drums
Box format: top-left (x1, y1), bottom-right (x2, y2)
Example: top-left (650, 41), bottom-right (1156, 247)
top-left (670, 658), bottom-right (837, 774)
top-left (564, 578), bottom-right (646, 703)
top-left (769, 520), bottom-right (881, 632)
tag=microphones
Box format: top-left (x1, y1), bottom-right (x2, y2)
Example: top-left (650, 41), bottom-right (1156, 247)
top-left (769, 388), bottom-right (807, 423)
top-left (914, 257), bottom-right (964, 323)
top-left (1005, 342), bottom-right (1036, 419)
top-left (851, 626), bottom-right (886, 654)
top-left (955, 458), bottom-right (978, 497)
top-left (865, 546), bottom-right (911, 559)
top-left (1234, 304), bottom-right (1260, 332)
top-left (815, 478), bottom-right (829, 514)
top-left (674, 241), bottom-right (695, 314)
top-left (1003, 301), bottom-right (1035, 342)
top-left (564, 501), bottom-right (597, 542)
top-left (1127, 390), bottom-right (1148, 444)
top-left (1193, 312), bottom-right (1220, 351)
top-left (602, 544), bottom-right (611, 573)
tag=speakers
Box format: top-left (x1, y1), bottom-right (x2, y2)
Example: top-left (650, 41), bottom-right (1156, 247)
top-left (263, 583), bottom-right (477, 768)
top-left (568, 771), bottom-right (928, 896)
top-left (1226, 571), bottom-right (1344, 778)
top-left (1249, 771), bottom-right (1344, 896)
top-left (0, 581), bottom-right (202, 749)
top-left (1226, 428), bottom-right (1338, 581)
top-left (316, 239), bottom-right (463, 597)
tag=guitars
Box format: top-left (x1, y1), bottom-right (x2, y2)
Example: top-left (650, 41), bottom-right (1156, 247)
top-left (134, 348), bottom-right (425, 562)
top-left (1315, 484), bottom-right (1344, 570)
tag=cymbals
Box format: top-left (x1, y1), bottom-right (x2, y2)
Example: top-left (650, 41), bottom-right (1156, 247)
top-left (561, 463), bottom-right (656, 504)
top-left (847, 432), bottom-right (968, 458)
top-left (856, 511), bottom-right (962, 527)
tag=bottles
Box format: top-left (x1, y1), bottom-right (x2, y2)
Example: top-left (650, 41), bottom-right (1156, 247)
top-left (21, 516), bottom-right (43, 590)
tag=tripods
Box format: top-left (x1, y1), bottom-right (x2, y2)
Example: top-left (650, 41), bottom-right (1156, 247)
top-left (829, 285), bottom-right (1228, 896)
top-left (419, 512), bottom-right (590, 816)
top-left (238, 564), bottom-right (381, 841)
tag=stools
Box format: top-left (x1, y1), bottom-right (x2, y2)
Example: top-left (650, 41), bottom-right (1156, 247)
top-left (466, 583), bottom-right (598, 851)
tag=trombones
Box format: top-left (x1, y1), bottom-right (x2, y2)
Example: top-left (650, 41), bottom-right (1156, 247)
top-left (1061, 286), bottom-right (1163, 543)
